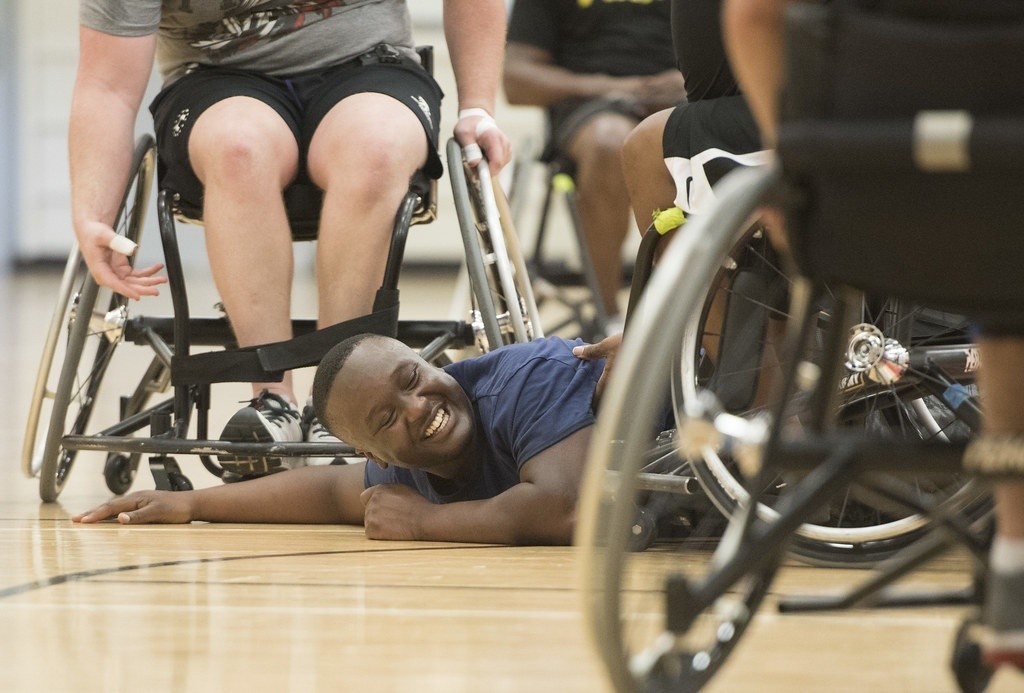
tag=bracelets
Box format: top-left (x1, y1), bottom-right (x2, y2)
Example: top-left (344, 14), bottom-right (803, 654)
top-left (456, 107), bottom-right (497, 136)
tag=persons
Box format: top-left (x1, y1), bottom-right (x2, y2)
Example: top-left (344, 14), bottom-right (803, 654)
top-left (503, 0), bottom-right (685, 314)
top-left (569, 0), bottom-right (1024, 661)
top-left (68, 0), bottom-right (512, 484)
top-left (69, 331), bottom-right (992, 546)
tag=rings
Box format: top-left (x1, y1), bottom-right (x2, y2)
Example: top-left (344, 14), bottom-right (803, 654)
top-left (111, 235), bottom-right (136, 257)
top-left (464, 143), bottom-right (483, 163)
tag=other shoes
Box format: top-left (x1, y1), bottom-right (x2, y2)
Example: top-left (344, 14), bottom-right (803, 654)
top-left (949, 615), bottom-right (1024, 693)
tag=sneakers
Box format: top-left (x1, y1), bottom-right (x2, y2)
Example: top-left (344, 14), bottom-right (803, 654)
top-left (299, 395), bottom-right (368, 466)
top-left (216, 387), bottom-right (305, 474)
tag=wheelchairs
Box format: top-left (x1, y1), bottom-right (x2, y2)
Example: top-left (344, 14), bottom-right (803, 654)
top-left (18, 46), bottom-right (543, 506)
top-left (575, 9), bottom-right (1024, 693)
top-left (446, 134), bottom-right (647, 365)
